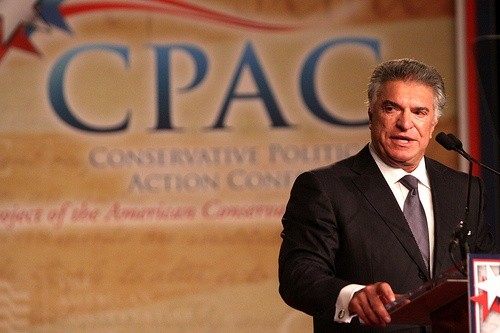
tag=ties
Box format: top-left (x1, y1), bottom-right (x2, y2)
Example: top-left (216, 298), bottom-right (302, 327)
top-left (399, 175), bottom-right (430, 278)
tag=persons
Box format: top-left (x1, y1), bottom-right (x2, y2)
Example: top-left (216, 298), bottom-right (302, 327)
top-left (277, 56), bottom-right (499, 333)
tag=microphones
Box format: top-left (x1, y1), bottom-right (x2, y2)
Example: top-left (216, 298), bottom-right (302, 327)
top-left (435, 132), bottom-right (473, 222)
top-left (446, 133), bottom-right (500, 175)
